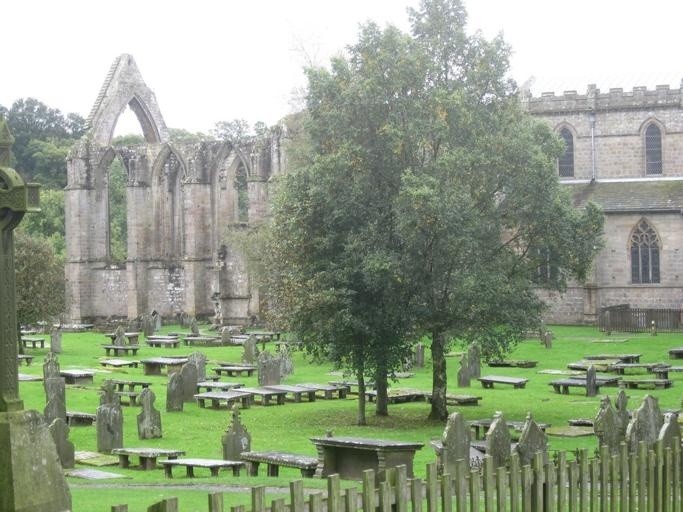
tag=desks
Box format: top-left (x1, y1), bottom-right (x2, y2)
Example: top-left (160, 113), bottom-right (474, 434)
top-left (310, 437), bottom-right (426, 488)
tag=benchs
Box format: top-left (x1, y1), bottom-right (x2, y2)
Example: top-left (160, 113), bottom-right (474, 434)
top-left (102, 346), bottom-right (139, 356)
top-left (547, 354), bottom-right (673, 395)
top-left (100, 360), bottom-right (141, 368)
top-left (104, 333), bottom-right (281, 349)
top-left (668, 348), bottom-right (683, 360)
top-left (111, 448), bottom-right (186, 470)
top-left (59, 369), bottom-right (94, 385)
top-left (487, 359), bottom-right (539, 367)
top-left (18, 354), bottom-right (35, 366)
top-left (141, 356), bottom-right (189, 376)
top-left (240, 452), bottom-right (317, 478)
top-left (364, 387), bottom-right (483, 406)
top-left (470, 418), bottom-right (552, 441)
top-left (477, 376), bottom-right (529, 390)
top-left (67, 411), bottom-right (98, 427)
top-left (158, 458), bottom-right (245, 478)
top-left (194, 380), bottom-right (359, 409)
top-left (429, 439), bottom-right (520, 472)
top-left (21, 338), bottom-right (45, 348)
top-left (105, 379), bottom-right (152, 407)
top-left (274, 342), bottom-right (303, 351)
top-left (210, 362), bottom-right (259, 376)
